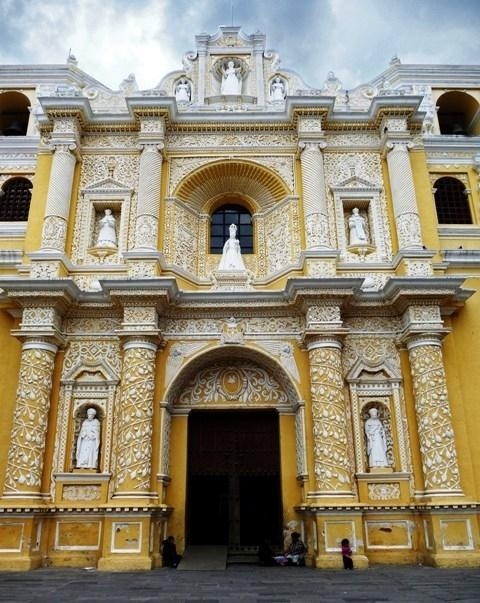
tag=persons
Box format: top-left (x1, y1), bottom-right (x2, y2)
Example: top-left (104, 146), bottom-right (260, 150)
top-left (218, 222), bottom-right (244, 270)
top-left (278, 529), bottom-right (308, 566)
top-left (75, 406), bottom-right (101, 470)
top-left (272, 76), bottom-right (285, 99)
top-left (365, 408), bottom-right (390, 466)
top-left (95, 207), bottom-right (118, 242)
top-left (349, 205), bottom-right (369, 243)
top-left (341, 537), bottom-right (354, 570)
top-left (220, 61), bottom-right (243, 95)
top-left (176, 77), bottom-right (189, 100)
top-left (159, 534), bottom-right (184, 567)
